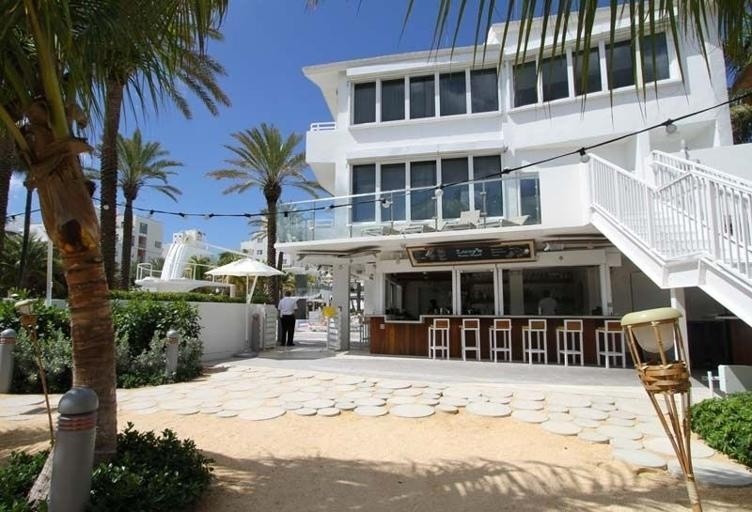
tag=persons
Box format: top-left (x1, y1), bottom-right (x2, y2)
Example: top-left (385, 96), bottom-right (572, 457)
top-left (277, 290), bottom-right (298, 347)
top-left (538, 290), bottom-right (559, 316)
top-left (427, 298), bottom-right (440, 314)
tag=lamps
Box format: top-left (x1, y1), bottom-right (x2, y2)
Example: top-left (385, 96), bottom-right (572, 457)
top-left (432, 187), bottom-right (445, 198)
top-left (663, 121), bottom-right (676, 136)
top-left (146, 210), bottom-right (155, 219)
top-left (322, 204), bottom-right (335, 214)
top-left (203, 213), bottom-right (215, 221)
top-left (177, 212), bottom-right (189, 221)
top-left (379, 199), bottom-right (391, 209)
top-left (578, 150), bottom-right (591, 164)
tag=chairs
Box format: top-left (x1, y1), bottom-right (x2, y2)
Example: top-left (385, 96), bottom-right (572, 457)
top-left (357, 313), bottom-right (370, 347)
top-left (428, 319), bottom-right (625, 369)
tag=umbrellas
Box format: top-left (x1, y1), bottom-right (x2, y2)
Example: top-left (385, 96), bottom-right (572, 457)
top-left (203, 255), bottom-right (284, 348)
